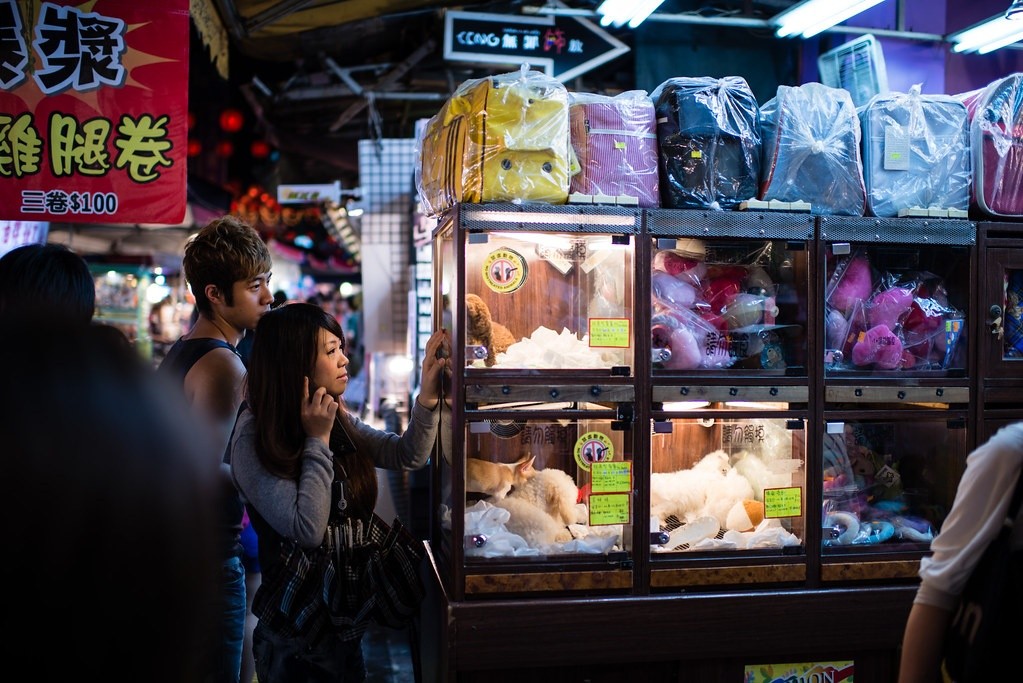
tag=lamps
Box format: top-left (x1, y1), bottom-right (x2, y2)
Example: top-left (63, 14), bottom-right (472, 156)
top-left (595, 0), bottom-right (1023, 58)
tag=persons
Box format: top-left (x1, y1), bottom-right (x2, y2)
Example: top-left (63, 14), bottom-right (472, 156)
top-left (223, 304), bottom-right (445, 683)
top-left (898, 421), bottom-right (1023, 683)
top-left (0, 241), bottom-right (216, 683)
top-left (141, 271), bottom-right (365, 378)
top-left (157, 213), bottom-right (273, 683)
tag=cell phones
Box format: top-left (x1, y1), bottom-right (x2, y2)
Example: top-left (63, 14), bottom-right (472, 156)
top-left (308, 383), bottom-right (357, 454)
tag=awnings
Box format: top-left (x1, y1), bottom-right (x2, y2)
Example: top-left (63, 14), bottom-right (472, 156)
top-left (46, 219), bottom-right (196, 268)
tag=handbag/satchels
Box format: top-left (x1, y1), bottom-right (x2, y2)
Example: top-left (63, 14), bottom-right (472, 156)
top-left (942, 531), bottom-right (1023, 683)
top-left (418, 70), bottom-right (1023, 224)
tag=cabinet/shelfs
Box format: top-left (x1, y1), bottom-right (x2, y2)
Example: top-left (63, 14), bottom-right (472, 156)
top-left (814, 214), bottom-right (982, 585)
top-left (978, 218), bottom-right (1023, 434)
top-left (419, 199), bottom-right (643, 602)
top-left (636, 205), bottom-right (817, 596)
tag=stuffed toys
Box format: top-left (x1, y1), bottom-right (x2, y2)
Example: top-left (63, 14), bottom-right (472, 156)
top-left (649, 449), bottom-right (768, 534)
top-left (466, 292), bottom-right (515, 368)
top-left (830, 248), bottom-right (960, 371)
top-left (467, 451), bottom-right (588, 548)
top-left (652, 237), bottom-right (781, 371)
top-left (824, 424), bottom-right (935, 545)
